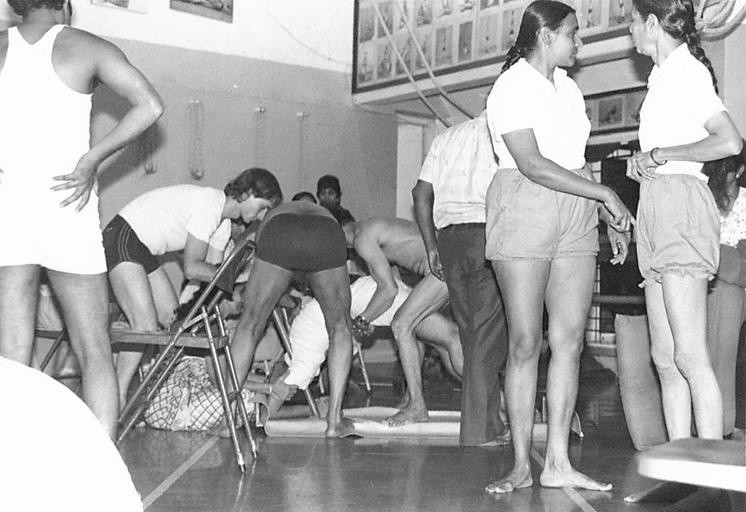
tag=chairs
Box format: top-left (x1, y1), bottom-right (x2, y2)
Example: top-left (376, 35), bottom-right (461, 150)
top-left (112, 237), bottom-right (260, 472)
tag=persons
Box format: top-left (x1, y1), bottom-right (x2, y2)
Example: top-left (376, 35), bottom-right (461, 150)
top-left (623, 1), bottom-right (746, 446)
top-left (414, 110), bottom-right (512, 449)
top-left (1, 1), bottom-right (165, 446)
top-left (484, 1), bottom-right (638, 493)
top-left (100, 169), bottom-right (464, 438)
